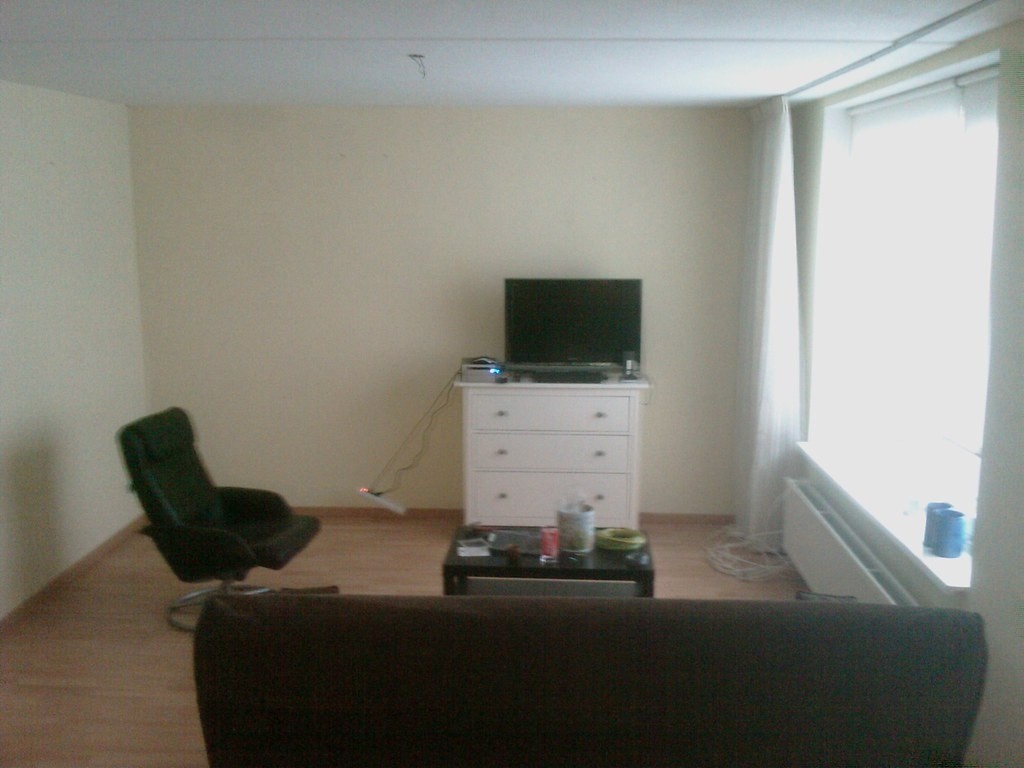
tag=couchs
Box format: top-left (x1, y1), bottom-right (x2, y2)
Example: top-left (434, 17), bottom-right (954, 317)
top-left (193, 594), bottom-right (987, 767)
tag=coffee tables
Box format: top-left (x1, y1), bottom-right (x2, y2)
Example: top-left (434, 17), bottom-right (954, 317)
top-left (442, 524), bottom-right (654, 599)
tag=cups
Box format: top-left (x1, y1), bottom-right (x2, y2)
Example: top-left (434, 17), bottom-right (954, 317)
top-left (540, 528), bottom-right (560, 563)
top-left (923, 502), bottom-right (965, 557)
top-left (556, 504), bottom-right (595, 552)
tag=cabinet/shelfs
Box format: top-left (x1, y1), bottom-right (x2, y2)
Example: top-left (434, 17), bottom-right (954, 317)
top-left (455, 368), bottom-right (650, 531)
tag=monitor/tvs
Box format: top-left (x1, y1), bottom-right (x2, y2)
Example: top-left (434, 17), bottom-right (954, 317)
top-left (505, 278), bottom-right (642, 383)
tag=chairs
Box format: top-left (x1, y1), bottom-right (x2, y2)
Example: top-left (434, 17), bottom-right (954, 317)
top-left (118, 405), bottom-right (320, 631)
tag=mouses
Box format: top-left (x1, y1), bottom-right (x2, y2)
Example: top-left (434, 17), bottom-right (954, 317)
top-left (472, 357), bottom-right (496, 364)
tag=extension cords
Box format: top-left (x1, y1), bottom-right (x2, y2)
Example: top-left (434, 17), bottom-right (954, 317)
top-left (362, 492), bottom-right (406, 516)
